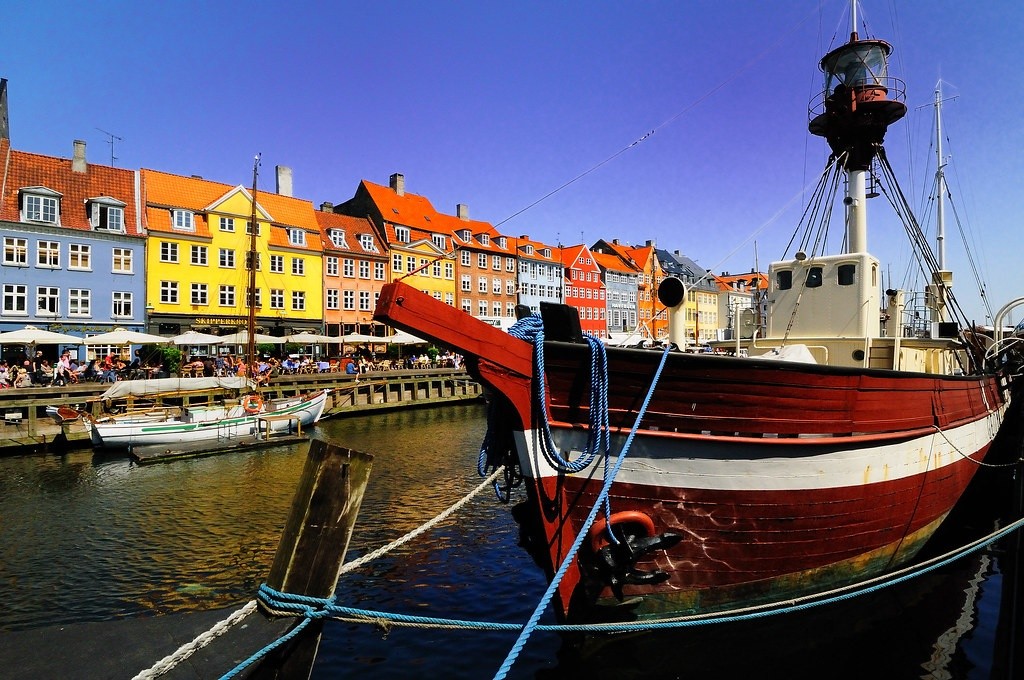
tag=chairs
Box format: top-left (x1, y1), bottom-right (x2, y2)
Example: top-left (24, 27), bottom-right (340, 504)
top-left (379, 359), bottom-right (446, 369)
top-left (66, 366), bottom-right (226, 381)
top-left (280, 361), bottom-right (340, 372)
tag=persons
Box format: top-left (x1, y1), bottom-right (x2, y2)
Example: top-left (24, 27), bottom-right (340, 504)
top-left (0, 351), bottom-right (467, 387)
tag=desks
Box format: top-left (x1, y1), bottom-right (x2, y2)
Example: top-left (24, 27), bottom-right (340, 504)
top-left (140, 368), bottom-right (153, 379)
top-left (299, 364), bottom-right (315, 373)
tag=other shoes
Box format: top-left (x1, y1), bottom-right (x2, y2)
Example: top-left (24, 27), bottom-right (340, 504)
top-left (42, 385), bottom-right (46, 387)
top-left (355, 380), bottom-right (360, 382)
top-left (264, 383), bottom-right (269, 386)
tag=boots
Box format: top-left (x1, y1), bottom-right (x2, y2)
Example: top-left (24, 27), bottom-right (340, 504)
top-left (6, 384), bottom-right (9, 389)
top-left (0, 383), bottom-right (2, 389)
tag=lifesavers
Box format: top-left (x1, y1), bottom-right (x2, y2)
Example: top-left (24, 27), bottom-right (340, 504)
top-left (244, 396), bottom-right (262, 413)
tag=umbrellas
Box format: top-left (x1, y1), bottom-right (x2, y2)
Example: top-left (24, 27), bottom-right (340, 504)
top-left (223, 329), bottom-right (287, 344)
top-left (0, 326), bottom-right (84, 370)
top-left (343, 332), bottom-right (391, 351)
top-left (391, 334), bottom-right (428, 358)
top-left (173, 331), bottom-right (224, 356)
top-left (287, 331), bottom-right (343, 357)
top-left (84, 327), bottom-right (173, 361)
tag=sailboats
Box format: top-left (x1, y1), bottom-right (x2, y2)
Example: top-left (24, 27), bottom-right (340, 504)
top-left (84, 154), bottom-right (329, 461)
top-left (373, 1), bottom-right (1014, 615)
